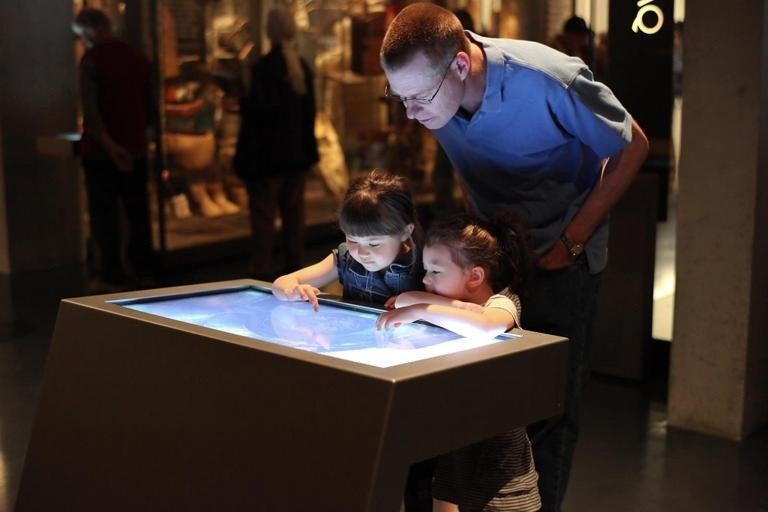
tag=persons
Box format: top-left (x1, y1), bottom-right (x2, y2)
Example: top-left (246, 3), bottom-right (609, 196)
top-left (68, 5), bottom-right (153, 287)
top-left (162, 89), bottom-right (240, 221)
top-left (271, 168), bottom-right (426, 313)
top-left (556, 16), bottom-right (605, 83)
top-left (381, 1), bottom-right (651, 512)
top-left (371, 211), bottom-right (545, 512)
top-left (228, 7), bottom-right (322, 284)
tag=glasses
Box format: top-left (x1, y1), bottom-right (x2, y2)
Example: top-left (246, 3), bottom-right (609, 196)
top-left (385, 54), bottom-right (458, 104)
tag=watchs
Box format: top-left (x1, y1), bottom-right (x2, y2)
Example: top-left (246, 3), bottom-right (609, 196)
top-left (559, 231), bottom-right (584, 257)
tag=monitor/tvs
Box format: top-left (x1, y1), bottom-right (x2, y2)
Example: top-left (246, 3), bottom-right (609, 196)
top-left (107, 285), bottom-right (521, 369)
